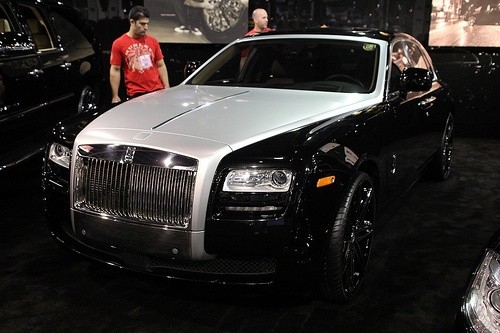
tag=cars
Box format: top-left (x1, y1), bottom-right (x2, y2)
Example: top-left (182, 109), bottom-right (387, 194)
top-left (40, 28), bottom-right (456, 305)
top-left (452, 227), bottom-right (500, 332)
top-left (428, 45), bottom-right (499, 139)
top-left (2, 2), bottom-right (100, 155)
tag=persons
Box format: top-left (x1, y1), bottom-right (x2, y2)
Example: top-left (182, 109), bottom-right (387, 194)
top-left (108, 6), bottom-right (170, 104)
top-left (239, 8), bottom-right (276, 74)
